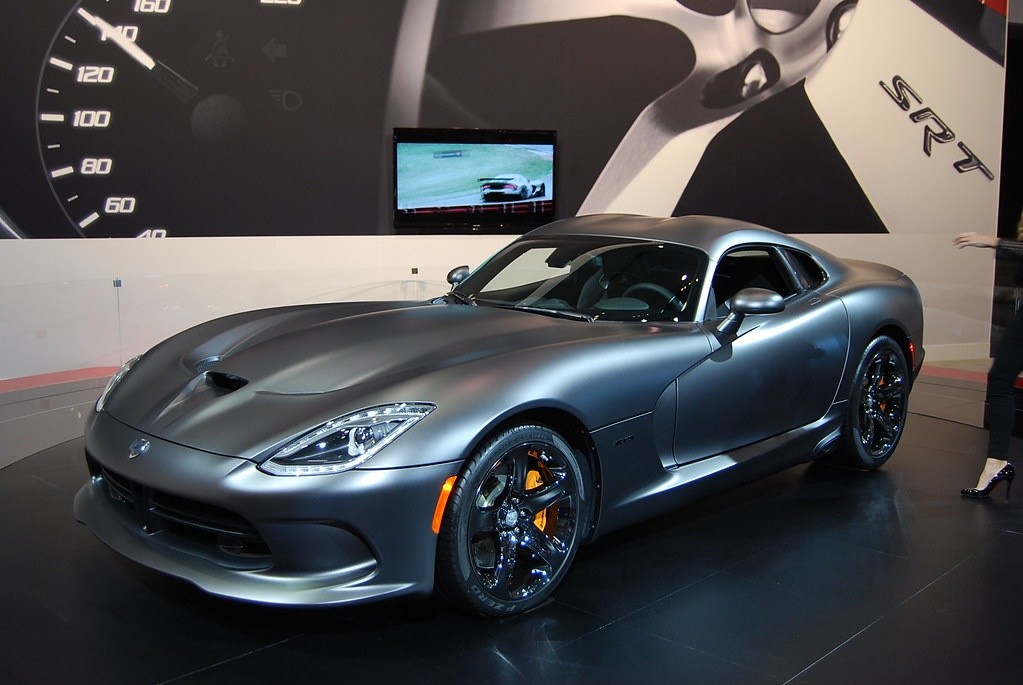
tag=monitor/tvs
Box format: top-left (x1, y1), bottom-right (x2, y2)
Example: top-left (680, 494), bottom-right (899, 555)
top-left (393, 127), bottom-right (558, 234)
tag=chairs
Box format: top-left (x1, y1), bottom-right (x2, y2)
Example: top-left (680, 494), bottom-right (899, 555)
top-left (576, 261), bottom-right (645, 310)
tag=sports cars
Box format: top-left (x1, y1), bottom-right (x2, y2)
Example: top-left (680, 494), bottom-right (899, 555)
top-left (475, 174), bottom-right (546, 202)
top-left (71, 213), bottom-right (925, 636)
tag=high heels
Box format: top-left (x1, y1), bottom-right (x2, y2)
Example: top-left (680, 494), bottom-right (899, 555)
top-left (960, 462), bottom-right (1016, 504)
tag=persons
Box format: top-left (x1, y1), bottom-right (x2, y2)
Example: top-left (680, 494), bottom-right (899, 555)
top-left (952, 230), bottom-right (1023, 498)
